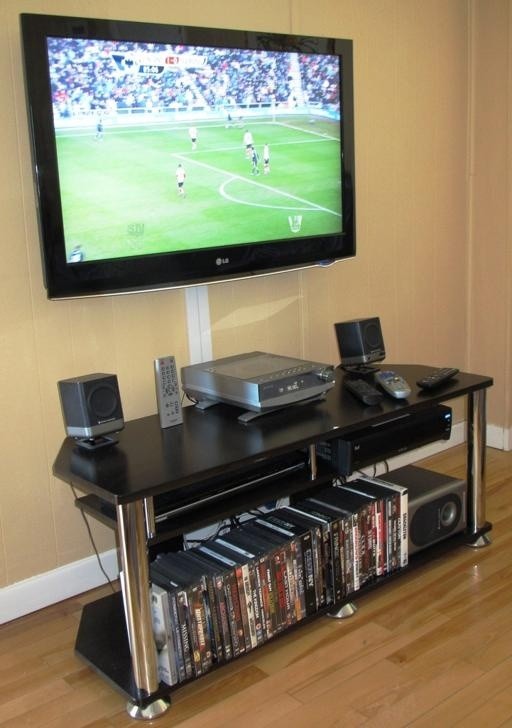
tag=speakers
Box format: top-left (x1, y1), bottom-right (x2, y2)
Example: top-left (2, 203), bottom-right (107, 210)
top-left (57, 373), bottom-right (125, 449)
top-left (334, 318), bottom-right (386, 376)
top-left (374, 464), bottom-right (469, 556)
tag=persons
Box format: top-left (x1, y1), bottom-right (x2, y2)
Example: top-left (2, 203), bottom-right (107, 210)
top-left (172, 162), bottom-right (189, 198)
top-left (67, 243), bottom-right (85, 262)
top-left (188, 124), bottom-right (199, 150)
top-left (241, 130), bottom-right (254, 159)
top-left (40, 33), bottom-right (344, 130)
top-left (250, 146), bottom-right (261, 176)
top-left (262, 143), bottom-right (271, 176)
top-left (94, 119), bottom-right (103, 140)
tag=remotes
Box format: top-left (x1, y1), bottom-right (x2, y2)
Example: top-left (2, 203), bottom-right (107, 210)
top-left (417, 367), bottom-right (459, 390)
top-left (153, 355), bottom-right (185, 430)
top-left (346, 377), bottom-right (383, 405)
top-left (373, 370), bottom-right (412, 399)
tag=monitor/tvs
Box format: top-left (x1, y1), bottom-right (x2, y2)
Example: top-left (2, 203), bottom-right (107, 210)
top-left (20, 12), bottom-right (356, 302)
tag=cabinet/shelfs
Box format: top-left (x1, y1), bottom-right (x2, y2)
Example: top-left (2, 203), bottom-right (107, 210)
top-left (55, 362), bottom-right (497, 720)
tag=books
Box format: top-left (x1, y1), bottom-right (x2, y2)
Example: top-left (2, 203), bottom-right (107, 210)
top-left (119, 472), bottom-right (411, 690)
